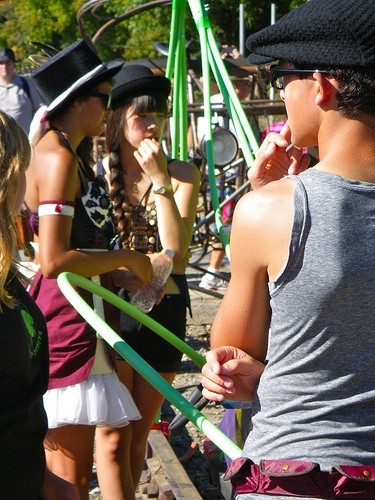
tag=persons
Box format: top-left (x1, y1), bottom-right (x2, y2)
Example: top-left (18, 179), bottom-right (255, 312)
top-left (197, 90), bottom-right (236, 289)
top-left (95, 65), bottom-right (200, 495)
top-left (0, 111), bottom-right (49, 500)
top-left (19, 35), bottom-right (143, 500)
top-left (0, 41), bottom-right (42, 138)
top-left (199, 0), bottom-right (374, 499)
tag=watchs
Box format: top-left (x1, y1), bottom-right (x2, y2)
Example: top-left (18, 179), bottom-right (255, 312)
top-left (149, 182), bottom-right (176, 198)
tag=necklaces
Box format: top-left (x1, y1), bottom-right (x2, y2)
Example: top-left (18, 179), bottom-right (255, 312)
top-left (118, 159), bottom-right (146, 195)
top-left (53, 123), bottom-right (89, 178)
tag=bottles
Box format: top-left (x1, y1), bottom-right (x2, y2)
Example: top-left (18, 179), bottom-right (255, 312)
top-left (130, 248), bottom-right (177, 312)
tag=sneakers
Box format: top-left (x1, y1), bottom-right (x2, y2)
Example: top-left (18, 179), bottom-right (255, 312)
top-left (198, 274), bottom-right (229, 290)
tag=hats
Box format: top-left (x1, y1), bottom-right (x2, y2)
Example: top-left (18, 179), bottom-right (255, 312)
top-left (28, 39), bottom-right (124, 119)
top-left (0, 48), bottom-right (16, 61)
top-left (246, 0), bottom-right (375, 65)
top-left (110, 65), bottom-right (171, 110)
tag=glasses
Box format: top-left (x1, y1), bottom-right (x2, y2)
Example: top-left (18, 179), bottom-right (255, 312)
top-left (83, 89), bottom-right (112, 110)
top-left (269, 65), bottom-right (336, 90)
